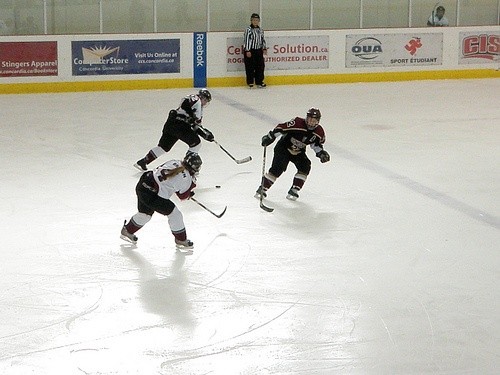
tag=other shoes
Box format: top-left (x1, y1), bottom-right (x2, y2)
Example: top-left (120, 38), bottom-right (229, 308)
top-left (258, 83), bottom-right (266, 88)
top-left (248, 84), bottom-right (254, 89)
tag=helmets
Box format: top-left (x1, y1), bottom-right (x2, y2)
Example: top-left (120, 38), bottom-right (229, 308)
top-left (436, 6), bottom-right (445, 12)
top-left (184, 149), bottom-right (202, 173)
top-left (305, 107), bottom-right (322, 131)
top-left (199, 88), bottom-right (212, 103)
top-left (250, 13), bottom-right (260, 21)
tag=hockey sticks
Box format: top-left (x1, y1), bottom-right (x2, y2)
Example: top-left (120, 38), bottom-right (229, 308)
top-left (259, 142), bottom-right (274, 213)
top-left (213, 140), bottom-right (253, 165)
top-left (191, 197), bottom-right (227, 218)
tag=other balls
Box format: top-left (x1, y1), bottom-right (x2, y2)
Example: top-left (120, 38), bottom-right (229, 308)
top-left (215, 185), bottom-right (221, 188)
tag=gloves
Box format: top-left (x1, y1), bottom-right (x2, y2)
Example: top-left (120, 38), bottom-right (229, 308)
top-left (316, 150), bottom-right (330, 164)
top-left (261, 130), bottom-right (276, 147)
top-left (197, 125), bottom-right (215, 143)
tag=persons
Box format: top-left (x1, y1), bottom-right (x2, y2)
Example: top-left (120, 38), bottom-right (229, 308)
top-left (133, 88), bottom-right (211, 171)
top-left (243, 13), bottom-right (267, 88)
top-left (428, 6), bottom-right (448, 26)
top-left (121, 152), bottom-right (203, 248)
top-left (254, 109), bottom-right (330, 200)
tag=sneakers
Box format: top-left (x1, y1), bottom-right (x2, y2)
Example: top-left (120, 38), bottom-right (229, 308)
top-left (133, 158), bottom-right (149, 172)
top-left (254, 185), bottom-right (267, 200)
top-left (285, 187), bottom-right (299, 201)
top-left (120, 220), bottom-right (138, 245)
top-left (174, 238), bottom-right (194, 250)
